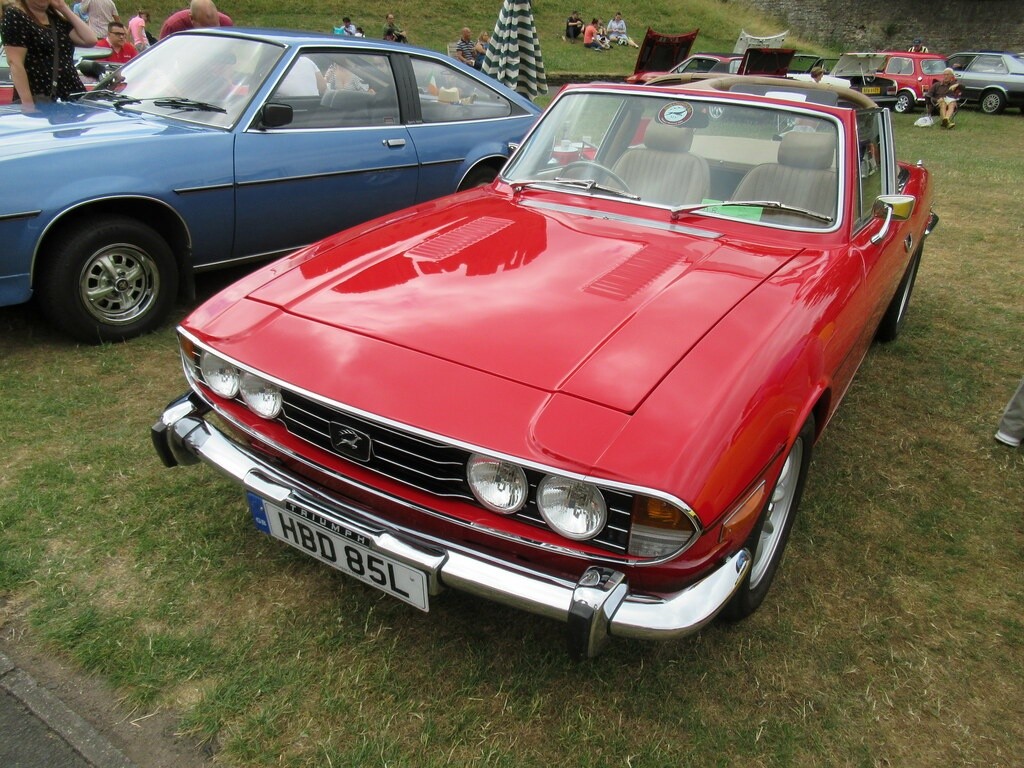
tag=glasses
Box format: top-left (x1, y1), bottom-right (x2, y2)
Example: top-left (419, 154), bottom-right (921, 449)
top-left (110, 32), bottom-right (125, 36)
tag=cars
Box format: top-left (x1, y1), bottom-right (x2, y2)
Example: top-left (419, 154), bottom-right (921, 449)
top-left (0, 24), bottom-right (541, 348)
top-left (923, 50), bottom-right (1024, 116)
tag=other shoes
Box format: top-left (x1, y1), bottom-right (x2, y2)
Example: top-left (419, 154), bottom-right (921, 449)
top-left (941, 117), bottom-right (949, 127)
top-left (947, 123), bottom-right (955, 129)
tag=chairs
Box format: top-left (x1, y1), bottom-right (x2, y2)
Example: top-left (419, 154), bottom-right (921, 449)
top-left (603, 117), bottom-right (712, 204)
top-left (926, 88), bottom-right (969, 125)
top-left (447, 42), bottom-right (458, 58)
top-left (730, 131), bottom-right (837, 228)
top-left (366, 85), bottom-right (400, 126)
top-left (305, 89), bottom-right (366, 128)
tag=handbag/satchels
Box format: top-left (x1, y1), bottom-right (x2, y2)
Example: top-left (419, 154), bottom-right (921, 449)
top-left (11, 94), bottom-right (52, 105)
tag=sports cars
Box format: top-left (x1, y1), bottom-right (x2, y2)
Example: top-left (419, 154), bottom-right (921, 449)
top-left (149, 22), bottom-right (959, 659)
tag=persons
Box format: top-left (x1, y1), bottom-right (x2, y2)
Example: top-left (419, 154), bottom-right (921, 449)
top-left (160, 0), bottom-right (234, 41)
top-left (807, 66), bottom-right (824, 83)
top-left (324, 59), bottom-right (370, 92)
top-left (382, 13), bottom-right (410, 44)
top-left (273, 55), bottom-right (327, 98)
top-left (0, 0), bottom-right (98, 105)
top-left (95, 21), bottom-right (138, 63)
top-left (607, 11), bottom-right (639, 49)
top-left (340, 17), bottom-right (366, 37)
top-left (128, 8), bottom-right (158, 52)
top-left (584, 17), bottom-right (614, 52)
top-left (566, 11), bottom-right (586, 44)
top-left (925, 67), bottom-right (962, 130)
top-left (903, 37), bottom-right (928, 68)
top-left (993, 378), bottom-right (1024, 447)
top-left (456, 27), bottom-right (490, 72)
top-left (74, 0), bottom-right (122, 39)
top-left (171, 51), bottom-right (233, 104)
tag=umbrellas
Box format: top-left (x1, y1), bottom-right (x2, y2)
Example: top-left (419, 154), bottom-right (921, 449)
top-left (479, 0), bottom-right (549, 102)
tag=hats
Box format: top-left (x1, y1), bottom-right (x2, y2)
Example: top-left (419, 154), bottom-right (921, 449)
top-left (811, 67), bottom-right (825, 75)
top-left (913, 39), bottom-right (921, 46)
top-left (190, 0), bottom-right (221, 27)
